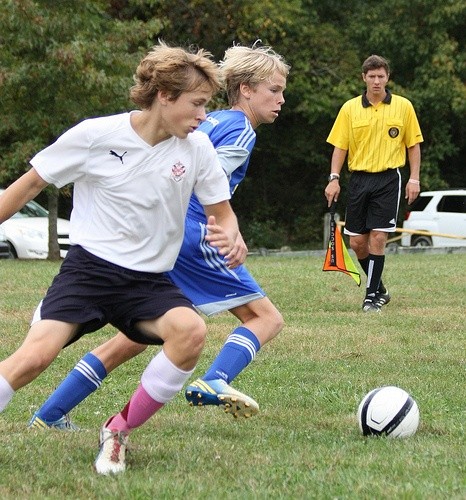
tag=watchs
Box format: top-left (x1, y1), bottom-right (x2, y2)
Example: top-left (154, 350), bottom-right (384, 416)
top-left (327, 175), bottom-right (339, 182)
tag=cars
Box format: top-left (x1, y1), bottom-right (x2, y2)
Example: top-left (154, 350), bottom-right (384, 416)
top-left (0, 189), bottom-right (72, 261)
top-left (401, 189), bottom-right (466, 248)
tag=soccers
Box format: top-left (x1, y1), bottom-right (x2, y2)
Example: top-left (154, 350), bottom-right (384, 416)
top-left (357, 385), bottom-right (419, 440)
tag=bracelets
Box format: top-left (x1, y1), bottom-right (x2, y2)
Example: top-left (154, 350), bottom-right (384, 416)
top-left (330, 173), bottom-right (340, 178)
top-left (409, 178), bottom-right (420, 185)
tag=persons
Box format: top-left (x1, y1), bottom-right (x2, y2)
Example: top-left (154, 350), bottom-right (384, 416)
top-left (25, 38), bottom-right (293, 433)
top-left (324, 55), bottom-right (420, 314)
top-left (0, 38), bottom-right (239, 476)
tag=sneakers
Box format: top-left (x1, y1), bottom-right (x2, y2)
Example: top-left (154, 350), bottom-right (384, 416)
top-left (92, 412), bottom-right (127, 476)
top-left (362, 288), bottom-right (390, 313)
top-left (185, 377), bottom-right (260, 418)
top-left (27, 410), bottom-right (89, 432)
